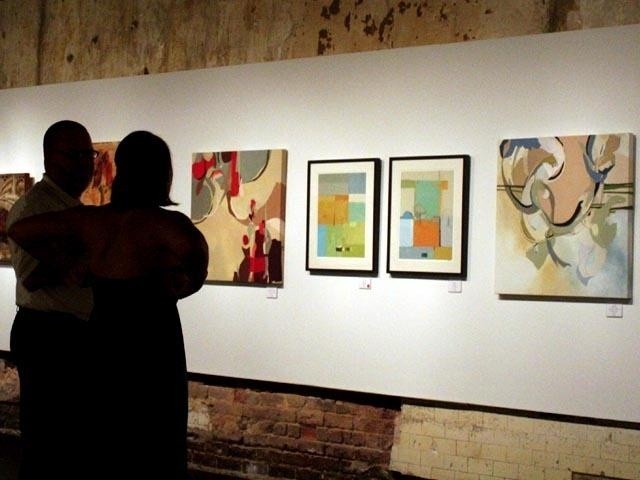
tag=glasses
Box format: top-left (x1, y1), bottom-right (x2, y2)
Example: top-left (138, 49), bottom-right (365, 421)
top-left (90, 151), bottom-right (99, 159)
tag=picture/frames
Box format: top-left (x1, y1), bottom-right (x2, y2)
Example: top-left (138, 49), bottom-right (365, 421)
top-left (306, 154), bottom-right (472, 281)
